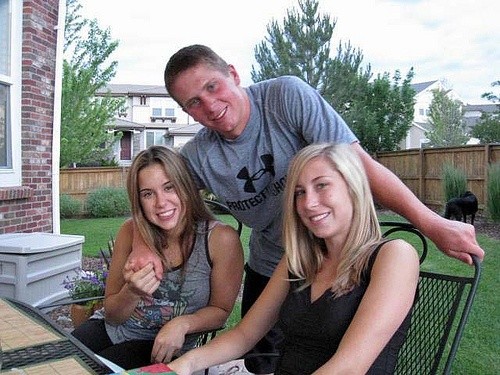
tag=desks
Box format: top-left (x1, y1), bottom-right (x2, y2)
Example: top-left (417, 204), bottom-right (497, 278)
top-left (0, 298), bottom-right (115, 375)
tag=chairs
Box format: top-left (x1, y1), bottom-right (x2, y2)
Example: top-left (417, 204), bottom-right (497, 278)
top-left (196, 198), bottom-right (482, 375)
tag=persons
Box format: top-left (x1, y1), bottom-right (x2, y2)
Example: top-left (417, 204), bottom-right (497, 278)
top-left (126, 44), bottom-right (485, 375)
top-left (167, 141), bottom-right (421, 375)
top-left (70, 146), bottom-right (243, 375)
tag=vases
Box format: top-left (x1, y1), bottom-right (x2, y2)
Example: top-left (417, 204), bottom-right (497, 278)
top-left (72, 306), bottom-right (93, 327)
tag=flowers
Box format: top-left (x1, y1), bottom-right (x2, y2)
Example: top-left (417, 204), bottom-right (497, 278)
top-left (63, 233), bottom-right (118, 304)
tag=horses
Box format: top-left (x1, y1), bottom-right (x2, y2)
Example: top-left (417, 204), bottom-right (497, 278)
top-left (445, 191), bottom-right (478, 225)
